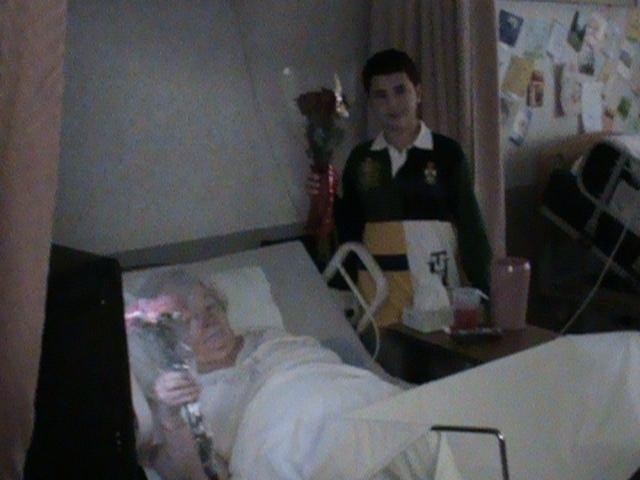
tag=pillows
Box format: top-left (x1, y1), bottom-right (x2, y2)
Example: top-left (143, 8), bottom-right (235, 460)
top-left (205, 266), bottom-right (286, 337)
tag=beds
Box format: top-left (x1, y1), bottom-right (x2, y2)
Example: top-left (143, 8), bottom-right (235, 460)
top-left (538, 136), bottom-right (640, 289)
top-left (120, 238), bottom-right (639, 480)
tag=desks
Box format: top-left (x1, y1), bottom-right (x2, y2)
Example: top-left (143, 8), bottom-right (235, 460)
top-left (378, 320), bottom-right (565, 372)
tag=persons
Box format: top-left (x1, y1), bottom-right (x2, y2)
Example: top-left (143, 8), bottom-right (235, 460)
top-left (305, 46), bottom-right (494, 374)
top-left (140, 272), bottom-right (342, 479)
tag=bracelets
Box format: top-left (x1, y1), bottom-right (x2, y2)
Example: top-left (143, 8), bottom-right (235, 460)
top-left (160, 423), bottom-right (185, 433)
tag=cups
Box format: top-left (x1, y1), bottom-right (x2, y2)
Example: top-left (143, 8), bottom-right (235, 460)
top-left (452, 286), bottom-right (482, 330)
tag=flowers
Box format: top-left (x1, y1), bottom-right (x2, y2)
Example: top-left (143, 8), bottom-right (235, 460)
top-left (293, 83), bottom-right (355, 264)
top-left (122, 291), bottom-right (232, 480)
